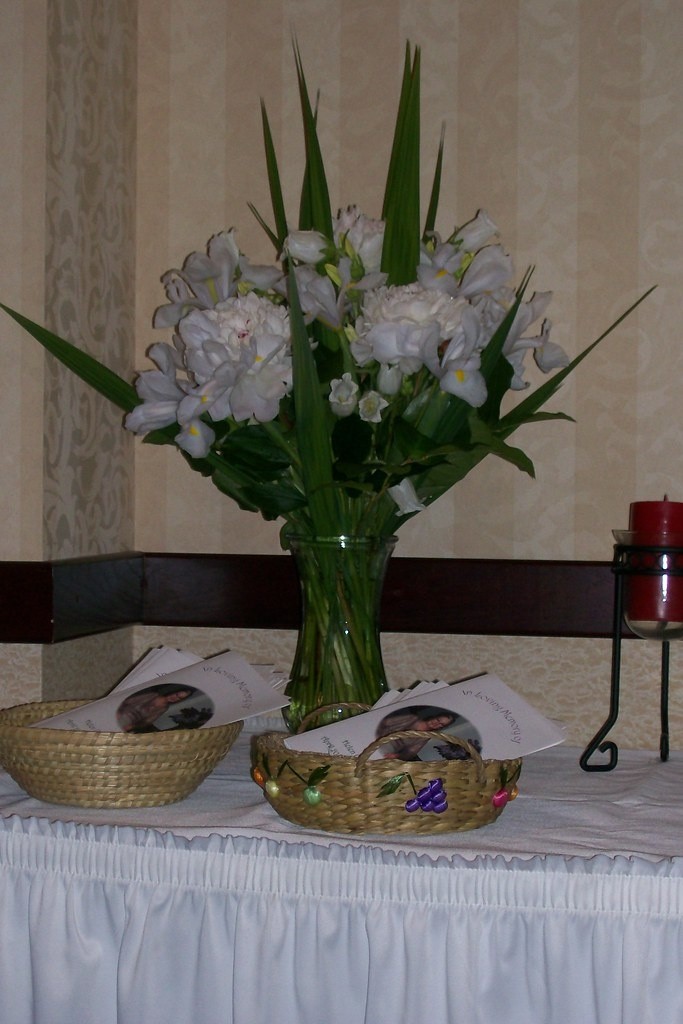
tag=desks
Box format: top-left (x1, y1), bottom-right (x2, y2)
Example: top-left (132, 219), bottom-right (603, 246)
top-left (0, 730), bottom-right (683, 1024)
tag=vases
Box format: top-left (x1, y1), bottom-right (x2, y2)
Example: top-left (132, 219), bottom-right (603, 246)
top-left (280, 534), bottom-right (400, 735)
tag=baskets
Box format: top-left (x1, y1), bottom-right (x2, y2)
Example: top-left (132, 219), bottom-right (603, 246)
top-left (0, 699), bottom-right (244, 809)
top-left (248, 702), bottom-right (522, 835)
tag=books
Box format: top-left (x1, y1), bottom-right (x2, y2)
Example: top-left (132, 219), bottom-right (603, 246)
top-left (283, 670), bottom-right (567, 761)
top-left (30, 645), bottom-right (291, 733)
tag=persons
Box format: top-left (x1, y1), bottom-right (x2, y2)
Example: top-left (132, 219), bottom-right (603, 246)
top-left (116, 688), bottom-right (193, 732)
top-left (380, 712), bottom-right (455, 760)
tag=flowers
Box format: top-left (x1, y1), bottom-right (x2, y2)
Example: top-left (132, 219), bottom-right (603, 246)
top-left (0, 18), bottom-right (657, 544)
